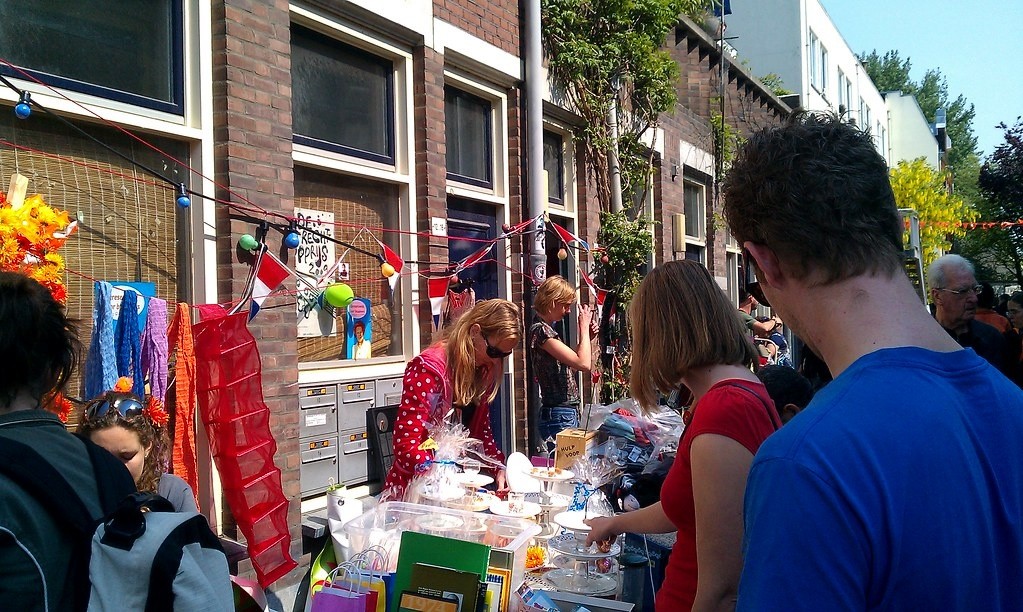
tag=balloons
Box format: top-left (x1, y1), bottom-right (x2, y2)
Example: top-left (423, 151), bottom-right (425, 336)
top-left (326, 282), bottom-right (354, 308)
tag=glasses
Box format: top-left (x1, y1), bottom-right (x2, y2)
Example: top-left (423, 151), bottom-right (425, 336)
top-left (1006, 311), bottom-right (1021, 317)
top-left (744, 237), bottom-right (774, 306)
top-left (85, 399), bottom-right (144, 420)
top-left (939, 285), bottom-right (983, 298)
top-left (481, 331), bottom-right (513, 358)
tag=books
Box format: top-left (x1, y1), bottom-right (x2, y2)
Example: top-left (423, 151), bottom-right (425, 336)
top-left (390, 531), bottom-right (560, 612)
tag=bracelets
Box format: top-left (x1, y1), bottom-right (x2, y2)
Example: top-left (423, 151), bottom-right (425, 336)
top-left (771, 318), bottom-right (777, 324)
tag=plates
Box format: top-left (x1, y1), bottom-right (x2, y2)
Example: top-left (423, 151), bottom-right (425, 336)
top-left (520, 493), bottom-right (573, 508)
top-left (506, 452), bottom-right (541, 493)
top-left (521, 466), bottom-right (576, 482)
top-left (489, 500), bottom-right (542, 519)
top-left (416, 483), bottom-right (466, 501)
top-left (548, 534), bottom-right (622, 562)
top-left (554, 511), bottom-right (604, 530)
top-left (452, 472), bottom-right (495, 487)
top-left (541, 569), bottom-right (617, 594)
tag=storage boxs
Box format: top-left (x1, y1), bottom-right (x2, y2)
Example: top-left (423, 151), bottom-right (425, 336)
top-left (344, 501), bottom-right (543, 595)
top-left (554, 427), bottom-right (598, 470)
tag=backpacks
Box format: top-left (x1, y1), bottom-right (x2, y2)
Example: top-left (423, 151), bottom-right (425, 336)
top-left (0, 435), bottom-right (234, 612)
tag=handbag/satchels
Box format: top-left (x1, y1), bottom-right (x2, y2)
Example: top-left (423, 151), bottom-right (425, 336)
top-left (310, 545), bottom-right (396, 612)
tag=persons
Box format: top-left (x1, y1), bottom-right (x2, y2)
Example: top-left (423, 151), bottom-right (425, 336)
top-left (77, 387), bottom-right (197, 513)
top-left (527, 275), bottom-right (600, 459)
top-left (352, 322), bottom-right (371, 359)
top-left (340, 263), bottom-right (347, 280)
top-left (927, 254), bottom-right (1023, 389)
top-left (582, 260), bottom-right (782, 612)
top-left (0, 273), bottom-right (137, 612)
top-left (720, 109), bottom-right (1023, 612)
top-left (736, 286), bottom-right (834, 424)
top-left (380, 298), bottom-right (522, 502)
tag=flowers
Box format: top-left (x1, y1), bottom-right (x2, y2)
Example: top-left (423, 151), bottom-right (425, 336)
top-left (145, 398), bottom-right (170, 427)
top-left (584, 370), bottom-right (602, 437)
top-left (0, 195), bottom-right (77, 425)
top-left (113, 376), bottom-right (133, 393)
